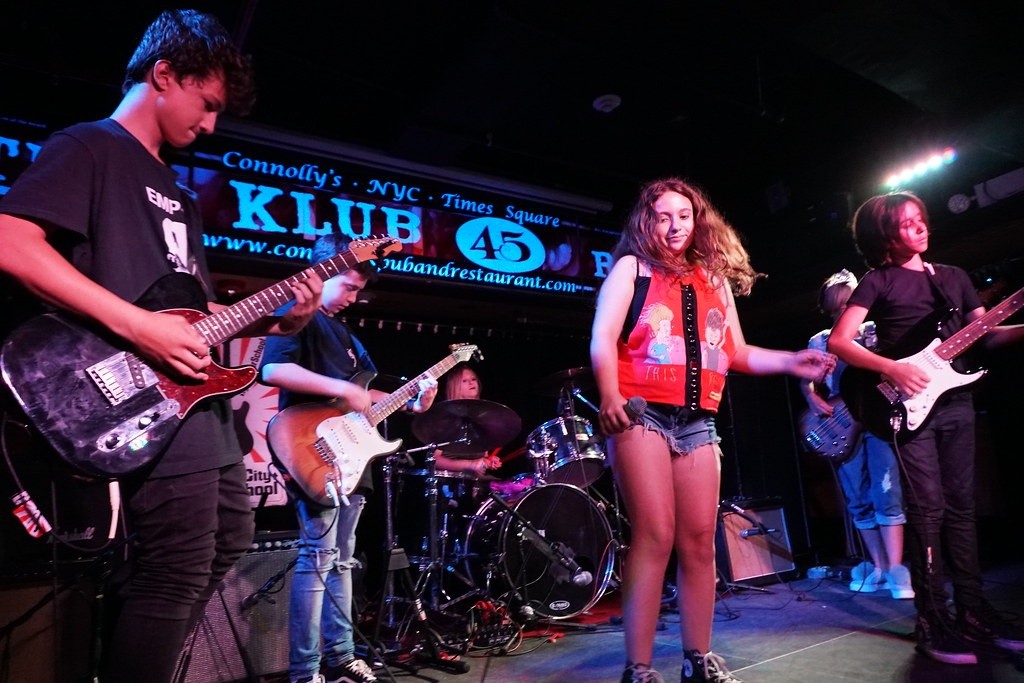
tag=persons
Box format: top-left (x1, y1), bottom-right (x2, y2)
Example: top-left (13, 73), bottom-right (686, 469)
top-left (256, 230), bottom-right (439, 683)
top-left (824, 190), bottom-right (1024, 663)
top-left (424, 366), bottom-right (503, 474)
top-left (801, 269), bottom-right (916, 599)
top-left (0, 10), bottom-right (325, 683)
top-left (591, 179), bottom-right (838, 683)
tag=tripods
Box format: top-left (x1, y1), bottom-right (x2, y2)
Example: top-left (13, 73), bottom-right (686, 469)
top-left (391, 420), bottom-right (598, 656)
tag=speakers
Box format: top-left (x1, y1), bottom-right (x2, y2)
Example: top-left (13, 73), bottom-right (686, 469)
top-left (712, 498), bottom-right (800, 591)
top-left (170, 528), bottom-right (301, 683)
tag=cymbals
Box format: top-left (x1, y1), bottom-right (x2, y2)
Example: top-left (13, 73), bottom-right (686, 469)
top-left (538, 366), bottom-right (596, 396)
top-left (411, 399), bottom-right (524, 455)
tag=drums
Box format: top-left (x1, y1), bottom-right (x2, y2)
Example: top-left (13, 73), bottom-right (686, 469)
top-left (383, 466), bottom-right (478, 563)
top-left (465, 483), bottom-right (615, 622)
top-left (526, 416), bottom-right (607, 490)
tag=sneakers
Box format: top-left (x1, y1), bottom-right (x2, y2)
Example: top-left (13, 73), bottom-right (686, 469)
top-left (319, 657), bottom-right (392, 683)
top-left (680, 649), bottom-right (742, 683)
top-left (850, 571), bottom-right (890, 592)
top-left (955, 602), bottom-right (1024, 650)
top-left (621, 663), bottom-right (664, 683)
top-left (890, 565), bottom-right (915, 599)
top-left (915, 614), bottom-right (979, 664)
top-left (290, 674), bottom-right (326, 683)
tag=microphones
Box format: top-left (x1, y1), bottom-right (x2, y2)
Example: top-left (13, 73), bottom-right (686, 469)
top-left (740, 527), bottom-right (780, 538)
top-left (579, 396), bottom-right (647, 452)
top-left (572, 571), bottom-right (594, 587)
top-left (242, 593), bottom-right (266, 610)
top-left (556, 388), bottom-right (565, 416)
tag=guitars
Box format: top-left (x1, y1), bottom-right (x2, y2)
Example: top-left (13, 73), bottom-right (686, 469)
top-left (0, 231), bottom-right (404, 476)
top-left (796, 391), bottom-right (866, 463)
top-left (267, 341), bottom-right (485, 507)
top-left (840, 286), bottom-right (1024, 444)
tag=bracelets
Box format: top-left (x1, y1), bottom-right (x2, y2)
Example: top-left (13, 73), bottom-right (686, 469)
top-left (407, 397), bottom-right (417, 415)
